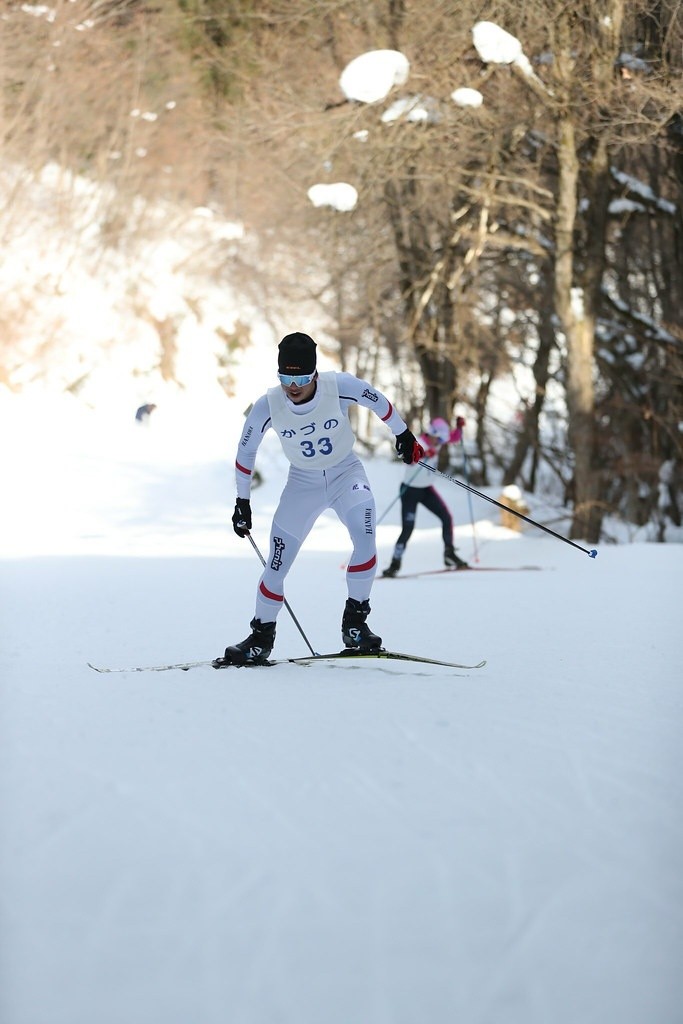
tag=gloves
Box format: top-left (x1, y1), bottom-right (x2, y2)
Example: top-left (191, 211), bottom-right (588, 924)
top-left (232, 497), bottom-right (252, 538)
top-left (395, 428), bottom-right (425, 464)
top-left (423, 449), bottom-right (435, 458)
top-left (456, 416), bottom-right (465, 429)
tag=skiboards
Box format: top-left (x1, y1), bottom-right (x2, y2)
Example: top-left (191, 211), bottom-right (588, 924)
top-left (85, 648), bottom-right (489, 675)
top-left (375, 565), bottom-right (542, 582)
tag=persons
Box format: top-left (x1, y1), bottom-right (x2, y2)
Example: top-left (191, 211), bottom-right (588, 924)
top-left (382, 416), bottom-right (468, 578)
top-left (224, 332), bottom-right (426, 661)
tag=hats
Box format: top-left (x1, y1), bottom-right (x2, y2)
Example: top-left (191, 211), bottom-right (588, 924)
top-left (428, 418), bottom-right (450, 438)
top-left (277, 332), bottom-right (317, 376)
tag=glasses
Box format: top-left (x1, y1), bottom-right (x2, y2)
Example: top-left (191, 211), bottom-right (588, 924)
top-left (278, 373), bottom-right (312, 387)
top-left (438, 437), bottom-right (446, 444)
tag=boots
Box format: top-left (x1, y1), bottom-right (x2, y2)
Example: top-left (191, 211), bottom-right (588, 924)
top-left (382, 557), bottom-right (401, 576)
top-left (341, 598), bottom-right (382, 650)
top-left (444, 546), bottom-right (467, 569)
top-left (225, 616), bottom-right (276, 661)
